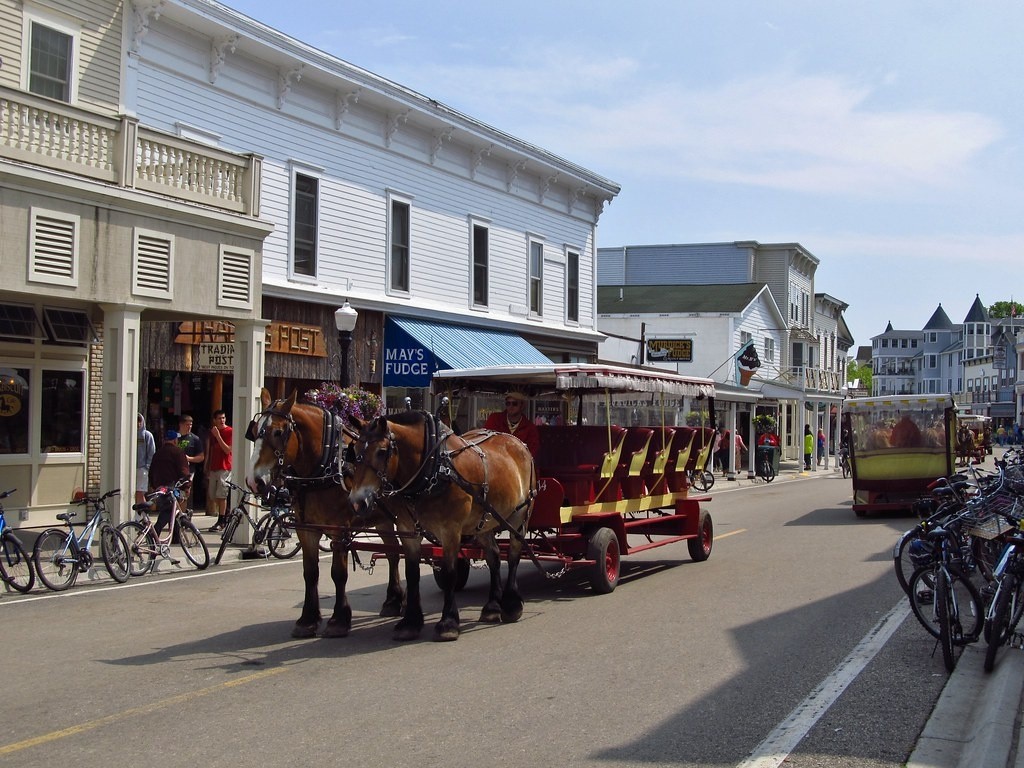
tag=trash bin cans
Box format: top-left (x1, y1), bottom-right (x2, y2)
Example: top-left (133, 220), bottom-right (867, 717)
top-left (756, 445), bottom-right (783, 476)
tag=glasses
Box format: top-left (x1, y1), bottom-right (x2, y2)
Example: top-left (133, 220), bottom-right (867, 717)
top-left (505, 401), bottom-right (518, 406)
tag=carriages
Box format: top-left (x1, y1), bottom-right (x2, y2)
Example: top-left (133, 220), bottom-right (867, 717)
top-left (957, 414), bottom-right (992, 467)
top-left (245, 363), bottom-right (717, 642)
top-left (842, 393), bottom-right (960, 517)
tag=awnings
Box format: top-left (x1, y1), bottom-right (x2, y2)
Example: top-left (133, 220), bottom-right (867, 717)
top-left (383, 314), bottom-right (556, 388)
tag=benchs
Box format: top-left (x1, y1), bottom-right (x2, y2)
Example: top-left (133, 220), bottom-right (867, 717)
top-left (533, 425), bottom-right (716, 506)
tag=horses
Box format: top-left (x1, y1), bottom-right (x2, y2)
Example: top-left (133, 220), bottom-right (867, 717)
top-left (244, 387), bottom-right (411, 638)
top-left (957, 424), bottom-right (975, 467)
top-left (341, 412), bottom-right (536, 643)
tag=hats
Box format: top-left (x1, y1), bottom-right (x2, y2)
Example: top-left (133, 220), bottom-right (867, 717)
top-left (818, 428), bottom-right (822, 431)
top-left (163, 430), bottom-right (182, 440)
top-left (505, 392), bottom-right (524, 400)
top-left (1000, 425), bottom-right (1002, 427)
top-left (725, 430), bottom-right (730, 435)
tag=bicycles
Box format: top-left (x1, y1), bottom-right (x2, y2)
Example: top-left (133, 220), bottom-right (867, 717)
top-left (30, 488), bottom-right (132, 591)
top-left (108, 481), bottom-right (210, 576)
top-left (215, 480), bottom-right (332, 565)
top-left (892, 446), bottom-right (1024, 672)
top-left (686, 470), bottom-right (715, 492)
top-left (839, 444), bottom-right (850, 479)
top-left (0, 488), bottom-right (35, 592)
top-left (758, 448), bottom-right (775, 483)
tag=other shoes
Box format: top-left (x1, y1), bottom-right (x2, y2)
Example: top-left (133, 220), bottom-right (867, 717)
top-left (737, 470), bottom-right (741, 474)
top-left (722, 474), bottom-right (725, 477)
top-left (209, 520), bottom-right (226, 531)
top-left (726, 474), bottom-right (728, 477)
top-left (804, 467), bottom-right (811, 470)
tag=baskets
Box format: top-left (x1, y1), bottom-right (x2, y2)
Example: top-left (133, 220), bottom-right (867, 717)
top-left (956, 493), bottom-right (1024, 540)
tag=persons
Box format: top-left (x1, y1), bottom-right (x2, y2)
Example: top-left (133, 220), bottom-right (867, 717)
top-left (804, 424), bottom-right (814, 469)
top-left (757, 429), bottom-right (780, 446)
top-left (817, 428), bottom-right (825, 465)
top-left (841, 429), bottom-right (848, 446)
top-left (997, 424), bottom-right (1006, 447)
top-left (890, 413), bottom-right (921, 447)
top-left (175, 414), bottom-right (204, 512)
top-left (1013, 423), bottom-right (1019, 441)
top-left (484, 391), bottom-right (541, 460)
top-left (204, 410), bottom-right (234, 533)
top-left (149, 430), bottom-right (190, 544)
top-left (713, 429), bottom-right (748, 477)
top-left (135, 412), bottom-right (155, 525)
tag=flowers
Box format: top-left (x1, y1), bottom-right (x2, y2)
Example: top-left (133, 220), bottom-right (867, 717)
top-left (988, 301), bottom-right (1024, 320)
top-left (303, 383), bottom-right (384, 421)
top-left (751, 414), bottom-right (777, 434)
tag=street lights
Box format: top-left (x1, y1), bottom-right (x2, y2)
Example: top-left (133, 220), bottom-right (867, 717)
top-left (335, 297), bottom-right (358, 390)
